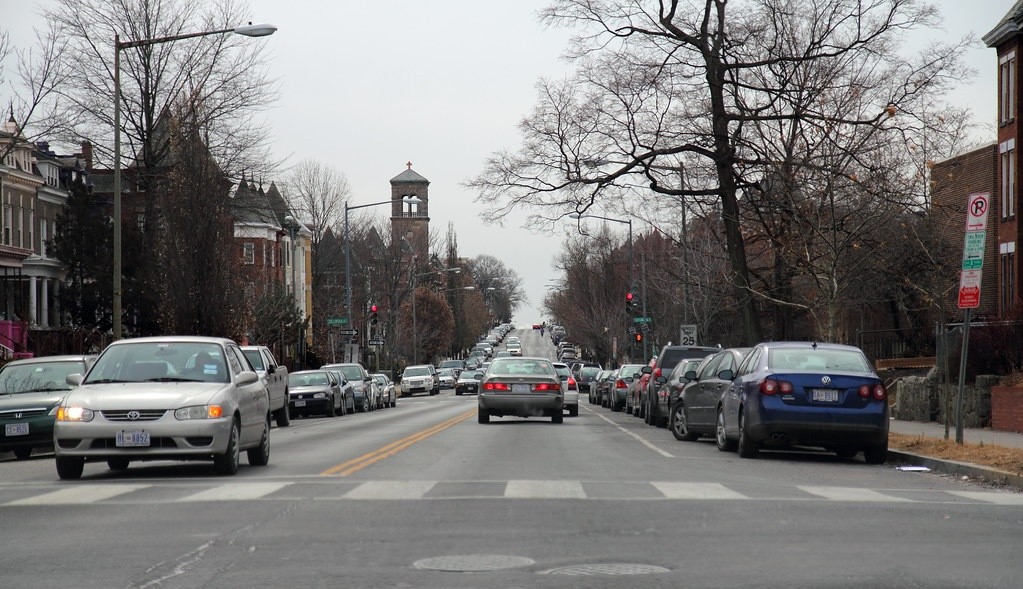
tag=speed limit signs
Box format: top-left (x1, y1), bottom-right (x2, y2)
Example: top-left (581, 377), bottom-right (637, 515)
top-left (680, 324), bottom-right (698, 347)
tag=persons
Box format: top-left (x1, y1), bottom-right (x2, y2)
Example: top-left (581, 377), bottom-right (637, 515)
top-left (540, 328), bottom-right (544, 338)
top-left (181, 352), bottom-right (225, 381)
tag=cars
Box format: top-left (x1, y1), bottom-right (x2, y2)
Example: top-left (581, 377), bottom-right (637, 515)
top-left (288, 324), bottom-right (522, 419)
top-left (0, 335), bottom-right (271, 480)
top-left (473, 357), bottom-right (579, 424)
top-left (656, 341), bottom-right (890, 464)
top-left (547, 322), bottom-right (657, 417)
top-left (533, 324), bottom-right (541, 330)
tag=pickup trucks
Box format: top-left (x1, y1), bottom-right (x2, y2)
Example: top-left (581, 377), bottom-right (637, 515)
top-left (185, 345), bottom-right (290, 427)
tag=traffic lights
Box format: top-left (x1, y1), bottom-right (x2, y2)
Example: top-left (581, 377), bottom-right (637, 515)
top-left (625, 292), bottom-right (634, 314)
top-left (635, 334), bottom-right (643, 342)
top-left (369, 304), bottom-right (379, 327)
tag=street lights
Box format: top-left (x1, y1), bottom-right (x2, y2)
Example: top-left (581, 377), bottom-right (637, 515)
top-left (412, 268), bottom-right (461, 365)
top-left (584, 158), bottom-right (689, 324)
top-left (345, 198), bottom-right (422, 344)
top-left (113, 22), bottom-right (277, 342)
top-left (569, 215), bottom-right (635, 365)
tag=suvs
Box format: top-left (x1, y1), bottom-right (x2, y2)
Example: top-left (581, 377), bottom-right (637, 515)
top-left (645, 342), bottom-right (724, 428)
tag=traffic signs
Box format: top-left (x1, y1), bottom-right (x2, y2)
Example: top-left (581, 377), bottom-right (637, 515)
top-left (340, 329), bottom-right (359, 336)
top-left (957, 270), bottom-right (982, 309)
top-left (369, 340), bottom-right (385, 346)
top-left (961, 231), bottom-right (986, 270)
